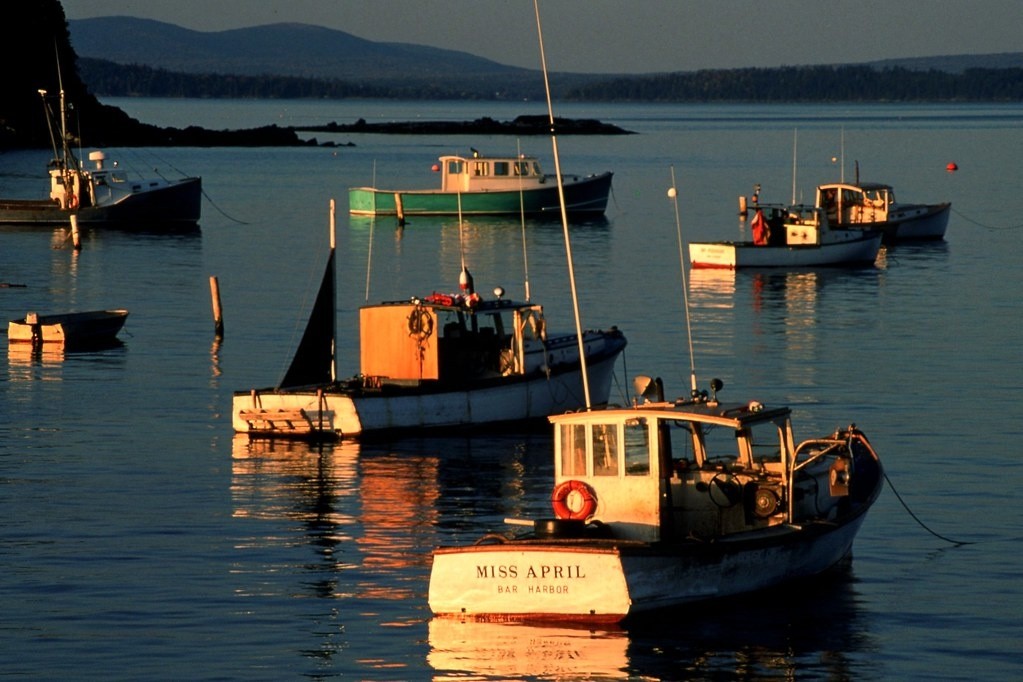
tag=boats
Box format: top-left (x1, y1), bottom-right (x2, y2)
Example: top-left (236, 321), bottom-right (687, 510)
top-left (423, 0), bottom-right (885, 619)
top-left (782, 126), bottom-right (956, 246)
top-left (342, 147), bottom-right (614, 223)
top-left (1, 88), bottom-right (206, 235)
top-left (683, 186), bottom-right (886, 281)
top-left (429, 617), bottom-right (795, 681)
top-left (7, 309), bottom-right (129, 357)
top-left (234, 188), bottom-right (631, 451)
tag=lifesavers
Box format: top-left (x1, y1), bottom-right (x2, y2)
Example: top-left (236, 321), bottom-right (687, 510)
top-left (552, 480), bottom-right (594, 521)
top-left (404, 310), bottom-right (430, 340)
top-left (68, 194), bottom-right (78, 208)
